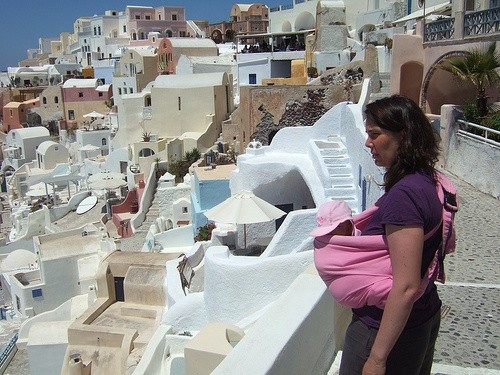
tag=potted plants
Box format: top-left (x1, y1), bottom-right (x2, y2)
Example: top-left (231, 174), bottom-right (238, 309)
top-left (141, 131), bottom-right (151, 142)
top-left (157, 62), bottom-right (168, 75)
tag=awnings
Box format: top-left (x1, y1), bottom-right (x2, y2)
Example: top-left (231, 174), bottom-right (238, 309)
top-left (392, 2), bottom-right (450, 26)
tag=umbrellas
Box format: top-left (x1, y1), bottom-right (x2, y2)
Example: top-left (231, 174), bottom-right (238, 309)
top-left (83, 110), bottom-right (105, 121)
top-left (4, 146), bottom-right (19, 158)
top-left (25, 181), bottom-right (55, 197)
top-left (86, 169), bottom-right (127, 205)
top-left (202, 191), bottom-right (288, 251)
top-left (413, 15), bottom-right (450, 25)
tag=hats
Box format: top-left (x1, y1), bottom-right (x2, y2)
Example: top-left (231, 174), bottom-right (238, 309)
top-left (308, 200), bottom-right (354, 236)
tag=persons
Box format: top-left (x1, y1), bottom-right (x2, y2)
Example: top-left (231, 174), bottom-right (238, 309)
top-left (30, 197), bottom-right (55, 211)
top-left (338, 93), bottom-right (451, 375)
top-left (308, 200), bottom-right (361, 237)
top-left (243, 40), bottom-right (269, 52)
top-left (279, 38), bottom-right (305, 51)
